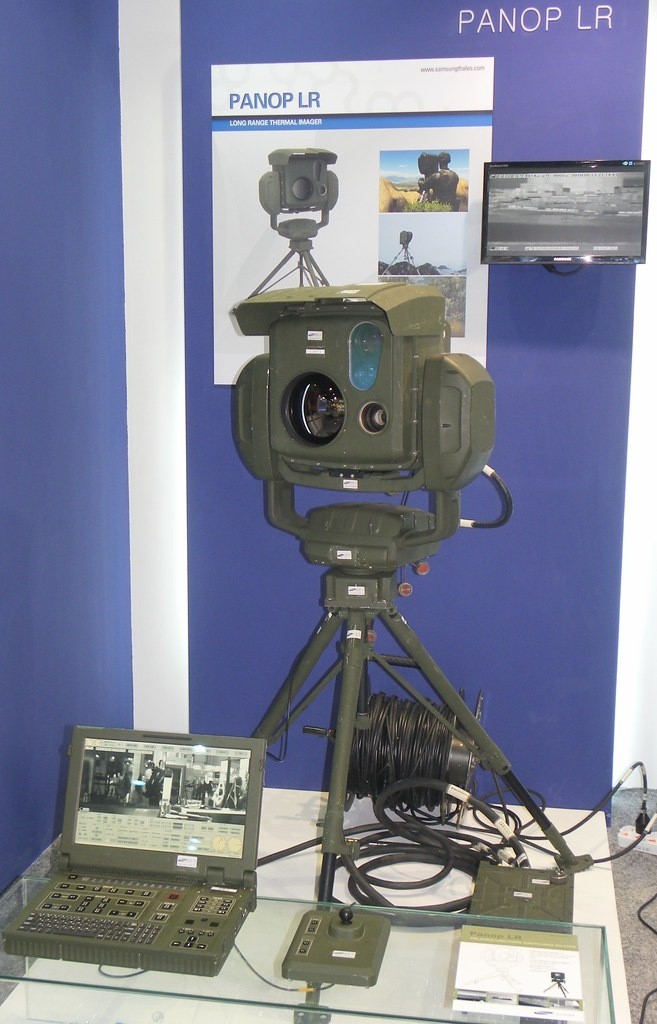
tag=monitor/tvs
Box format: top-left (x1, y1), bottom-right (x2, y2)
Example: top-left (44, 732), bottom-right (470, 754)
top-left (480, 160), bottom-right (651, 264)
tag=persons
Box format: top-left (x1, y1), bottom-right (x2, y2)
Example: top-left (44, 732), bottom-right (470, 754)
top-left (103, 757), bottom-right (244, 810)
top-left (417, 150), bottom-right (459, 211)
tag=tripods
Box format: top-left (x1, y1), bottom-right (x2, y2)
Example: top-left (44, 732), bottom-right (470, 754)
top-left (250, 568), bottom-right (595, 911)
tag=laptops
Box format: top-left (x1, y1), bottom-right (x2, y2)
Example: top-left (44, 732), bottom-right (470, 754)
top-left (4, 724), bottom-right (270, 976)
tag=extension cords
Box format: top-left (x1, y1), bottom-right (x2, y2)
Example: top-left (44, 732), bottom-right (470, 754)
top-left (618, 826), bottom-right (657, 855)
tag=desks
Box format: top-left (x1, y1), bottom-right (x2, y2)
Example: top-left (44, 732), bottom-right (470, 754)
top-left (160, 804), bottom-right (246, 825)
top-left (0, 788), bottom-right (633, 1024)
